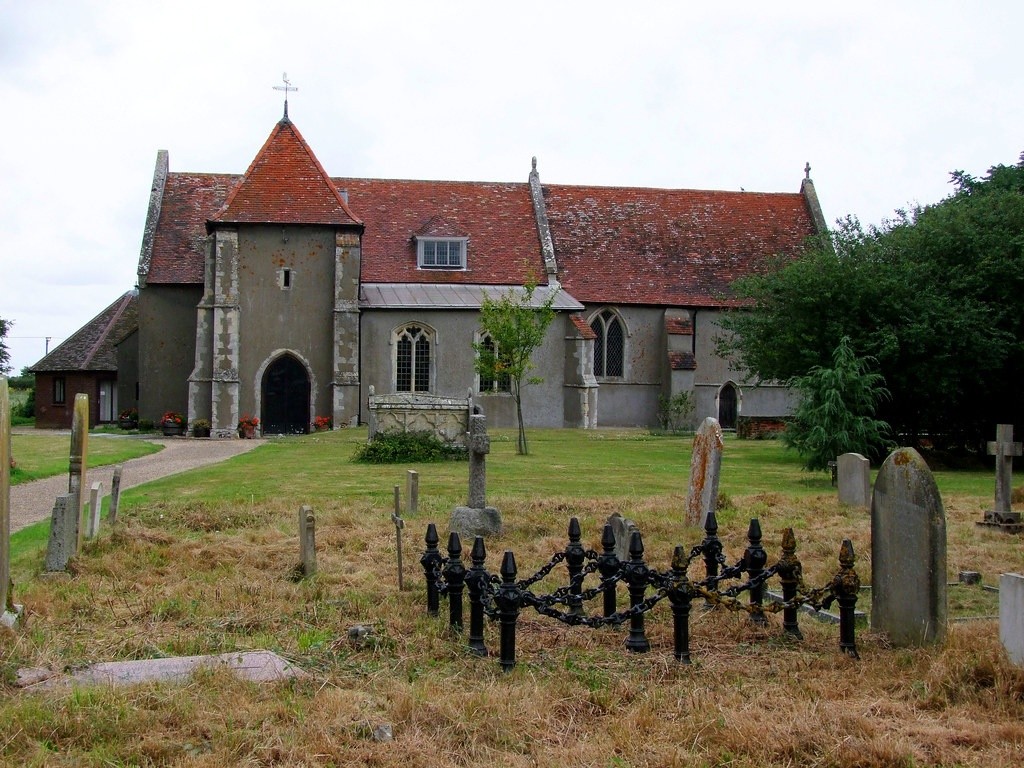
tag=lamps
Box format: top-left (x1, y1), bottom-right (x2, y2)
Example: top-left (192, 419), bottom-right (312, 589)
top-left (284, 237), bottom-right (289, 243)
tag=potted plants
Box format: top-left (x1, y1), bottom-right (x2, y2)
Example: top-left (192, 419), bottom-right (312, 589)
top-left (192, 419), bottom-right (211, 438)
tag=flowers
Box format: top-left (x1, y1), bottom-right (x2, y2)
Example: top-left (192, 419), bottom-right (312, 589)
top-left (309, 415), bottom-right (332, 428)
top-left (237, 417), bottom-right (259, 432)
top-left (159, 410), bottom-right (187, 426)
top-left (118, 406), bottom-right (137, 419)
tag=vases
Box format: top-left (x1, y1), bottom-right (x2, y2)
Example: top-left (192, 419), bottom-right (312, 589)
top-left (163, 422), bottom-right (184, 436)
top-left (120, 419), bottom-right (137, 429)
top-left (316, 425), bottom-right (329, 431)
top-left (243, 426), bottom-right (255, 438)
